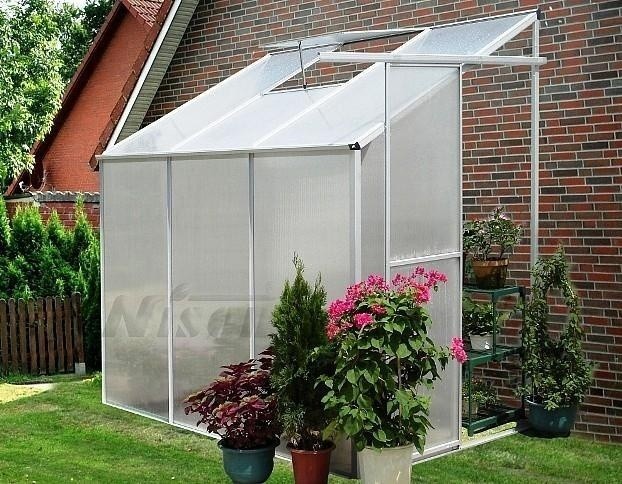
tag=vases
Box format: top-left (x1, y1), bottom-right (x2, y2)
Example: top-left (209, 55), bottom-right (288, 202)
top-left (357, 440), bottom-right (414, 483)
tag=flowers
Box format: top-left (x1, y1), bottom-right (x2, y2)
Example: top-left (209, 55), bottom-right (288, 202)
top-left (307, 266), bottom-right (468, 456)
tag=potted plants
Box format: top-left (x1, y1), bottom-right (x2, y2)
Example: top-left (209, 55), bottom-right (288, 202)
top-left (457, 201), bottom-right (600, 437)
top-left (182, 250), bottom-right (338, 484)
